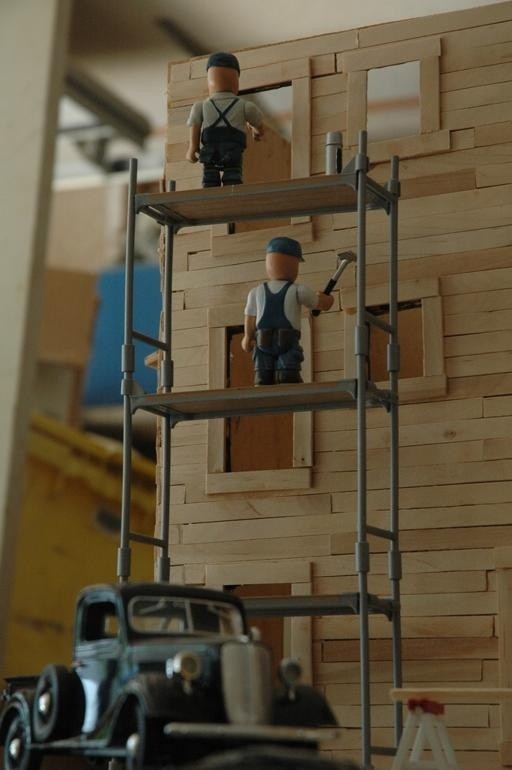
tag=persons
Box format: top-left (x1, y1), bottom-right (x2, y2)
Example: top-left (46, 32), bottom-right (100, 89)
top-left (241, 237), bottom-right (357, 385)
top-left (186, 53), bottom-right (265, 190)
